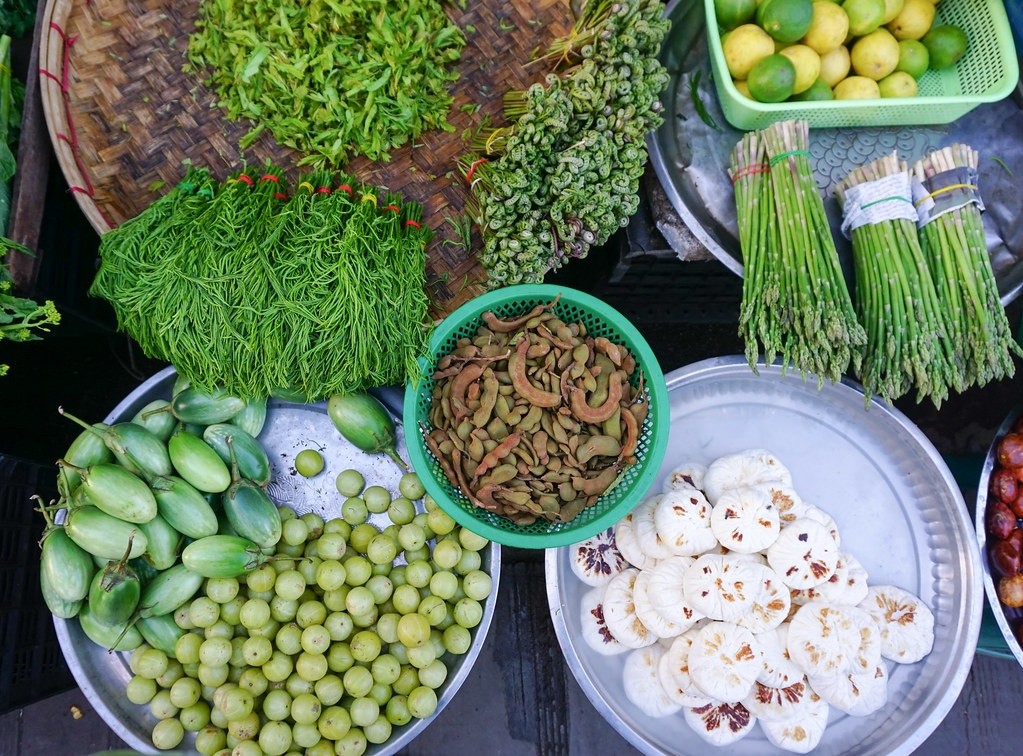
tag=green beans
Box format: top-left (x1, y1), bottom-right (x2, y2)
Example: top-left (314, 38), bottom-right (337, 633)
top-left (429, 298), bottom-right (650, 526)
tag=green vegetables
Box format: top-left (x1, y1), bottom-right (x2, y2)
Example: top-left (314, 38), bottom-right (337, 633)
top-left (86, 165), bottom-right (441, 401)
top-left (0, 0), bottom-right (61, 379)
top-left (452, 0), bottom-right (675, 289)
top-left (179, 0), bottom-right (480, 167)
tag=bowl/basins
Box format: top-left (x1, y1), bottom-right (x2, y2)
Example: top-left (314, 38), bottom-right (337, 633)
top-left (644, 2), bottom-right (1023, 313)
top-left (544, 353), bottom-right (984, 754)
top-left (42, 366), bottom-right (501, 756)
top-left (975, 400), bottom-right (1023, 679)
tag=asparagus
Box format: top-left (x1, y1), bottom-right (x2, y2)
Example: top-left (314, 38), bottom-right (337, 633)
top-left (726, 118), bottom-right (1023, 411)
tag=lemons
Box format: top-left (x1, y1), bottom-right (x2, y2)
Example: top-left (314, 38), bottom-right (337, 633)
top-left (713, 0), bottom-right (969, 103)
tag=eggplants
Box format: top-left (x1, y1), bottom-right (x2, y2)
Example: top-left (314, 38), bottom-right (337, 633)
top-left (34, 374), bottom-right (411, 653)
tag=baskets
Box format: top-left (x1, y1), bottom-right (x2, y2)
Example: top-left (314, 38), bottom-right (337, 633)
top-left (705, 0), bottom-right (1021, 130)
top-left (403, 282), bottom-right (671, 550)
